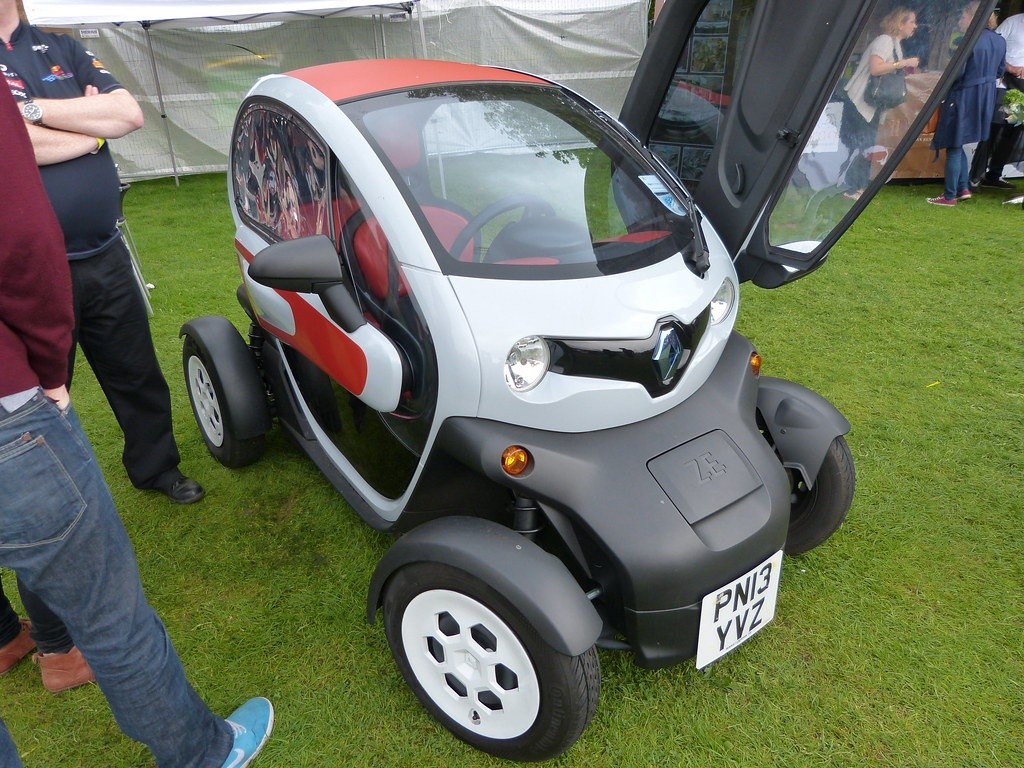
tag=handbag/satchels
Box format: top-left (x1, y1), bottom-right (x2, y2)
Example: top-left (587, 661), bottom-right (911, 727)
top-left (863, 72), bottom-right (907, 109)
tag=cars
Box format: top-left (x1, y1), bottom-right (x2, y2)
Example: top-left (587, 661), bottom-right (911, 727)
top-left (178, 1), bottom-right (999, 763)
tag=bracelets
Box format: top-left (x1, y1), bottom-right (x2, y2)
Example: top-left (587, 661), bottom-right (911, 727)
top-left (89, 137), bottom-right (106, 155)
top-left (892, 61), bottom-right (902, 69)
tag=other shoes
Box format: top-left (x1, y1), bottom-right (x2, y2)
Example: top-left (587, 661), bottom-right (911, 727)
top-left (843, 189), bottom-right (864, 200)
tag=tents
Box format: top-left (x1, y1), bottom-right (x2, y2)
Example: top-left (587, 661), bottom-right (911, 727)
top-left (22, 0), bottom-right (647, 203)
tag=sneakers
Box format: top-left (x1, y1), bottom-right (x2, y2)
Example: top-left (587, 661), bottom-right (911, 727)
top-left (221, 696), bottom-right (275, 768)
top-left (0, 619), bottom-right (36, 674)
top-left (957, 189), bottom-right (972, 201)
top-left (926, 193), bottom-right (957, 206)
top-left (981, 176), bottom-right (1017, 189)
top-left (969, 179), bottom-right (982, 193)
top-left (32, 644), bottom-right (96, 692)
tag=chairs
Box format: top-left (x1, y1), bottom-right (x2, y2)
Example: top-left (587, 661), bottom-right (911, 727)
top-left (339, 194), bottom-right (479, 296)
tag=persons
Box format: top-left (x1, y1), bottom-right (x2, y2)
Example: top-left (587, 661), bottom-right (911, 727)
top-left (839, 0), bottom-right (1024, 206)
top-left (0, 0), bottom-right (276, 768)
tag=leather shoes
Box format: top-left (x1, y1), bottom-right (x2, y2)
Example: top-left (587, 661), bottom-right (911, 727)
top-left (153, 468), bottom-right (206, 503)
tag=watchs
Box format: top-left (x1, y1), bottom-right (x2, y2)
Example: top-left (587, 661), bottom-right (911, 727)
top-left (22, 97), bottom-right (45, 128)
